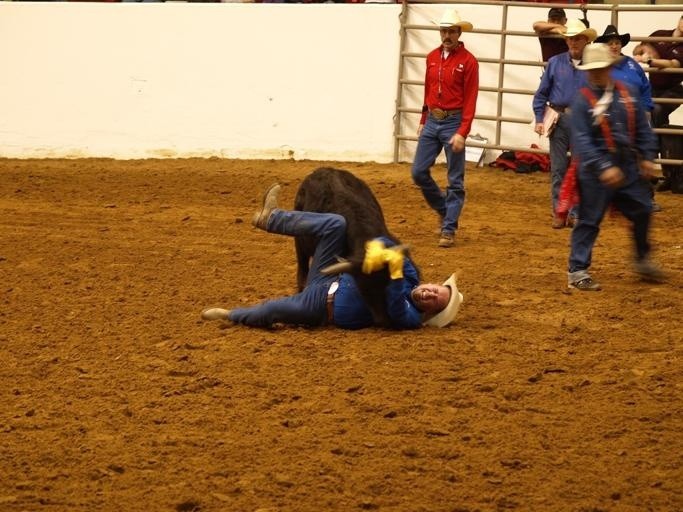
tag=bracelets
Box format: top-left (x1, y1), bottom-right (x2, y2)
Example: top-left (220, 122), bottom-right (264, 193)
top-left (647, 56), bottom-right (652, 64)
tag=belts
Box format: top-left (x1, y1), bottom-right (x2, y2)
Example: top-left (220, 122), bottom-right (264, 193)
top-left (425, 107), bottom-right (461, 120)
top-left (549, 103), bottom-right (568, 113)
top-left (326, 275), bottom-right (339, 324)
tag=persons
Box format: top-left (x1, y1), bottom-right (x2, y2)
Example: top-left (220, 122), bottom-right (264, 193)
top-left (531, 8), bottom-right (569, 81)
top-left (632, 14), bottom-right (681, 194)
top-left (200, 181), bottom-right (466, 331)
top-left (409, 8), bottom-right (479, 248)
top-left (531, 19), bottom-right (600, 231)
top-left (567, 42), bottom-right (668, 293)
top-left (593, 25), bottom-right (662, 215)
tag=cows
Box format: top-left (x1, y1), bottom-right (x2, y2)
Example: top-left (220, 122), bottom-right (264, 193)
top-left (291, 165), bottom-right (411, 328)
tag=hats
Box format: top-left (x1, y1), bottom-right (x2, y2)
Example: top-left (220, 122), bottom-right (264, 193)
top-left (422, 270), bottom-right (465, 330)
top-left (556, 18), bottom-right (631, 47)
top-left (571, 42), bottom-right (626, 72)
top-left (547, 7), bottom-right (566, 22)
top-left (429, 8), bottom-right (474, 31)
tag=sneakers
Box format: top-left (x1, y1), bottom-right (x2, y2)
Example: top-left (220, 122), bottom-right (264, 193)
top-left (199, 306), bottom-right (233, 325)
top-left (568, 277), bottom-right (603, 291)
top-left (650, 199), bottom-right (662, 213)
top-left (636, 263), bottom-right (664, 282)
top-left (252, 182), bottom-right (284, 233)
top-left (551, 210), bottom-right (579, 230)
top-left (432, 217), bottom-right (456, 247)
